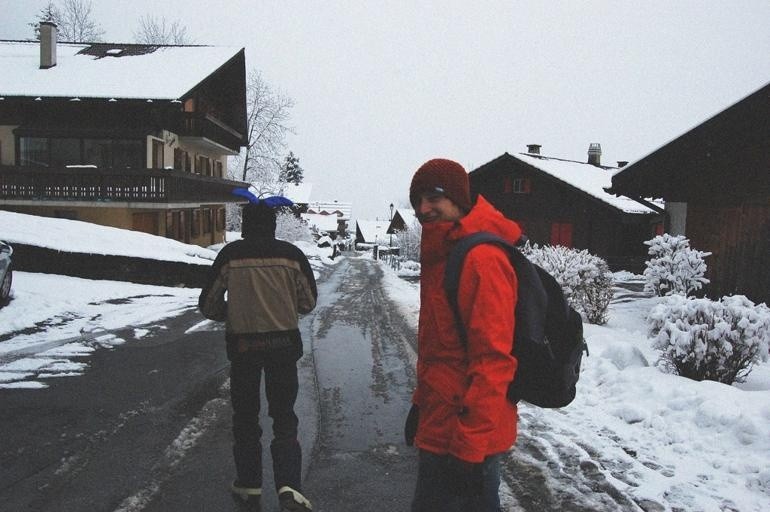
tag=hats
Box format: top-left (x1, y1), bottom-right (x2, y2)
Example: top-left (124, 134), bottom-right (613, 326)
top-left (410, 158), bottom-right (471, 212)
top-left (232, 188), bottom-right (293, 230)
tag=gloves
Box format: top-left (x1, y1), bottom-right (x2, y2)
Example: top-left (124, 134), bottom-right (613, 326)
top-left (404, 403), bottom-right (418, 445)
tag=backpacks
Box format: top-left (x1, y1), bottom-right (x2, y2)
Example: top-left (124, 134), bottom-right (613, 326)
top-left (444, 232), bottom-right (589, 408)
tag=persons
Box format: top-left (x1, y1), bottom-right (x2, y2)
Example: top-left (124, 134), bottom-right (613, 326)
top-left (404, 159), bottom-right (523, 512)
top-left (199, 190), bottom-right (317, 511)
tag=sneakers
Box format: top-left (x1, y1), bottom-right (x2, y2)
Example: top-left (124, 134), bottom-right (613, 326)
top-left (275, 481), bottom-right (313, 512)
top-left (232, 479), bottom-right (262, 495)
